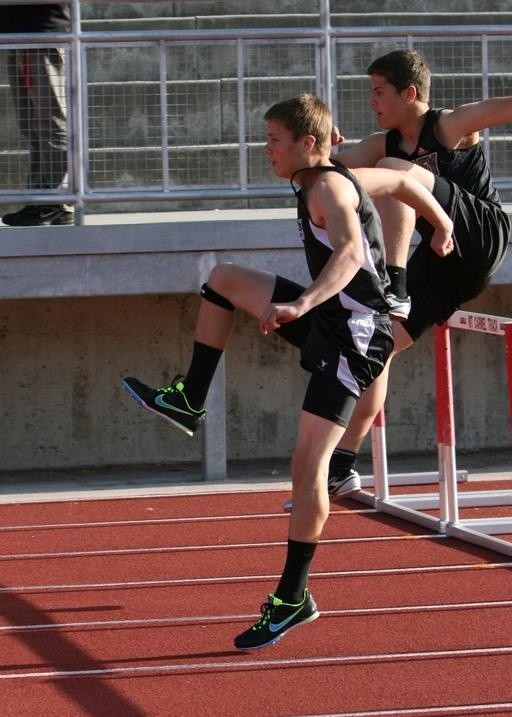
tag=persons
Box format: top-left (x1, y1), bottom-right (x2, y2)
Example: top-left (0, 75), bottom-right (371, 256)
top-left (282, 47), bottom-right (512, 512)
top-left (0, 3), bottom-right (76, 226)
top-left (119, 94), bottom-right (455, 651)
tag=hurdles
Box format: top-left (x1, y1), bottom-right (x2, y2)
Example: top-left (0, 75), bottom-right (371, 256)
top-left (348, 308), bottom-right (512, 557)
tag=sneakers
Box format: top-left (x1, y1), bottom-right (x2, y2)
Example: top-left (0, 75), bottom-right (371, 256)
top-left (2, 205), bottom-right (75, 226)
top-left (385, 294), bottom-right (411, 321)
top-left (283, 468), bottom-right (362, 513)
top-left (235, 588), bottom-right (320, 650)
top-left (121, 374), bottom-right (208, 440)
